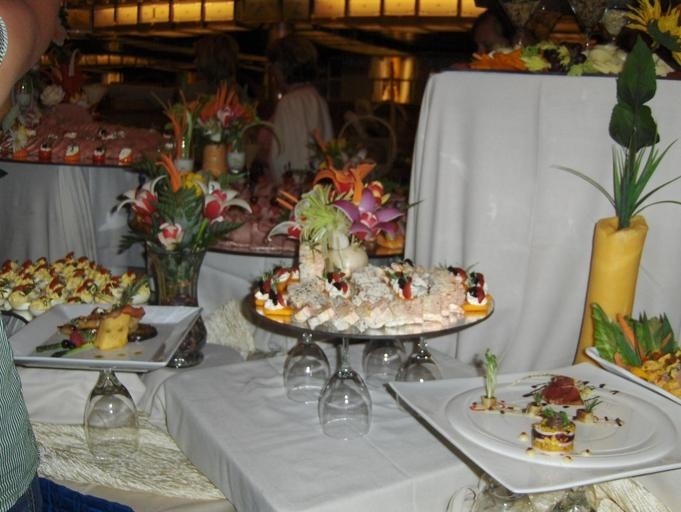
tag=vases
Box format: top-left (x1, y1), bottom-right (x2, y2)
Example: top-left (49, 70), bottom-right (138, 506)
top-left (143, 246), bottom-right (209, 367)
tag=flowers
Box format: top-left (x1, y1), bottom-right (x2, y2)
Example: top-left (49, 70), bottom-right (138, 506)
top-left (109, 155), bottom-right (244, 258)
top-left (147, 74), bottom-right (286, 175)
top-left (284, 161), bottom-right (394, 249)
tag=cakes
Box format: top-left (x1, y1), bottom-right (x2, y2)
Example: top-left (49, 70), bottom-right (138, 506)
top-left (0, 256), bottom-right (150, 310)
top-left (222, 178), bottom-right (313, 256)
top-left (533, 417), bottom-right (576, 454)
top-left (38, 143), bottom-right (133, 167)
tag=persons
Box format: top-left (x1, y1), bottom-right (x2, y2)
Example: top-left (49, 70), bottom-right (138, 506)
top-left (255, 31), bottom-right (335, 181)
top-left (169, 31), bottom-right (248, 101)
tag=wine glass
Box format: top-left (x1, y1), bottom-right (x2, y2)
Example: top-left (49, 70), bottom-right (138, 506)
top-left (500, 0), bottom-right (629, 58)
top-left (11, 78), bottom-right (34, 126)
top-left (82, 367), bottom-right (139, 463)
top-left (283, 330), bottom-right (447, 442)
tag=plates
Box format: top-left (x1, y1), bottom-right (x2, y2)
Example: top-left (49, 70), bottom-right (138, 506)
top-left (583, 346), bottom-right (681, 403)
top-left (383, 359), bottom-right (681, 495)
top-left (5, 302), bottom-right (204, 373)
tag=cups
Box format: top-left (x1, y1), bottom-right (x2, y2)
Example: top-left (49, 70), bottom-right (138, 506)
top-left (442, 472), bottom-right (597, 512)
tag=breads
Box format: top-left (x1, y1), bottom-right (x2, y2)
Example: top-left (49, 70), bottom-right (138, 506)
top-left (288, 269), bottom-right (467, 332)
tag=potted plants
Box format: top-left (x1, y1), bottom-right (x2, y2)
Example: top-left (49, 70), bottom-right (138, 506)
top-left (554, 122), bottom-right (681, 371)
